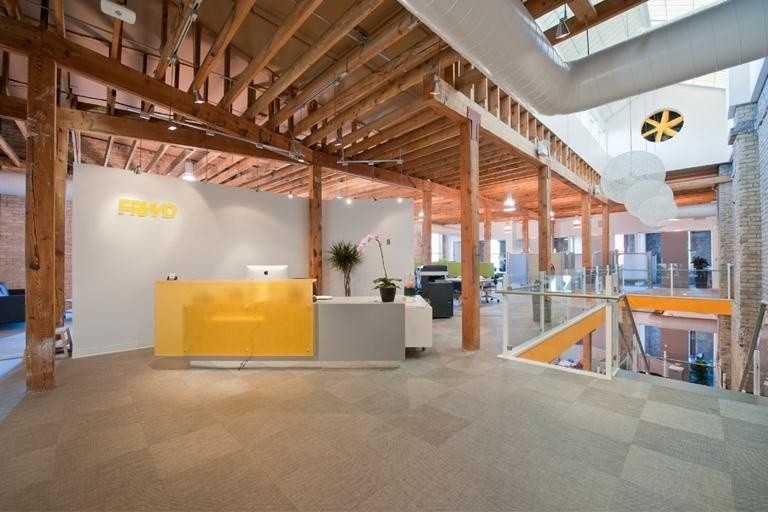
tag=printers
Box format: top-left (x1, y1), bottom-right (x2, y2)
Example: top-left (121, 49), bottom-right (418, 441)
top-left (416, 265), bottom-right (453, 290)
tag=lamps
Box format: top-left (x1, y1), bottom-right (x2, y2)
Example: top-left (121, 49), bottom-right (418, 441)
top-left (179, 158), bottom-right (200, 186)
top-left (555, 1), bottom-right (571, 39)
top-left (168, 0), bottom-right (205, 131)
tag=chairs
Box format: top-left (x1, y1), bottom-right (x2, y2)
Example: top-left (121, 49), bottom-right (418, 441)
top-left (0, 282), bottom-right (25, 324)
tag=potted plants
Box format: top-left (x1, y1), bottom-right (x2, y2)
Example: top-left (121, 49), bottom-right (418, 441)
top-left (691, 256), bottom-right (710, 288)
top-left (373, 277), bottom-right (403, 302)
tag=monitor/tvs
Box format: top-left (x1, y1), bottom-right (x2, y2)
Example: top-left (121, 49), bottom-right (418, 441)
top-left (246, 264), bottom-right (289, 280)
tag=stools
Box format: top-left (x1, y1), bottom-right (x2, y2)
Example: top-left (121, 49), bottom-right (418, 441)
top-left (55, 327), bottom-right (72, 358)
